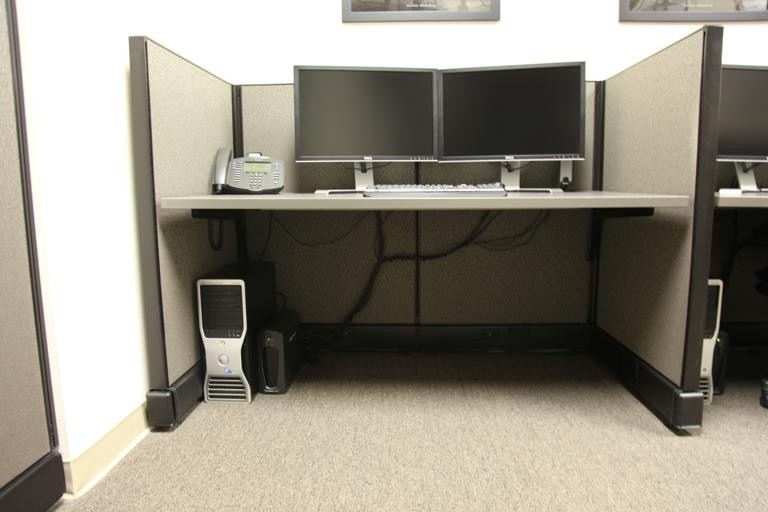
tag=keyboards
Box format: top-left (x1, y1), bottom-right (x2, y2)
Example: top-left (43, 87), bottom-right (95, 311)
top-left (364, 182), bottom-right (507, 197)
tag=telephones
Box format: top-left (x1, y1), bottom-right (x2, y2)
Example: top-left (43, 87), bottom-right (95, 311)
top-left (213, 147), bottom-right (285, 193)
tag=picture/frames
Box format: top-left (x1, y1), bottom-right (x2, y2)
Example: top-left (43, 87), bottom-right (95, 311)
top-left (618, 0), bottom-right (767, 24)
top-left (341, 0), bottom-right (500, 23)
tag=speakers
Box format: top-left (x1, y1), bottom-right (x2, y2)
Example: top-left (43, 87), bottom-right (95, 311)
top-left (714, 331), bottom-right (726, 394)
top-left (257, 309), bottom-right (300, 394)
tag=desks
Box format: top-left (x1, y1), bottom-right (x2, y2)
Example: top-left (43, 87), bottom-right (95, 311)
top-left (157, 187), bottom-right (689, 211)
top-left (716, 188), bottom-right (766, 208)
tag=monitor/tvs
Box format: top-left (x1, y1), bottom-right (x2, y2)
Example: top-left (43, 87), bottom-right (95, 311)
top-left (439, 62), bottom-right (586, 194)
top-left (714, 64), bottom-right (768, 197)
top-left (294, 65), bottom-right (438, 196)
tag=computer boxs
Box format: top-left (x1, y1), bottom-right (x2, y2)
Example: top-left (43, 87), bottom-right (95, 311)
top-left (195, 260), bottom-right (276, 404)
top-left (699, 278), bottom-right (723, 405)
